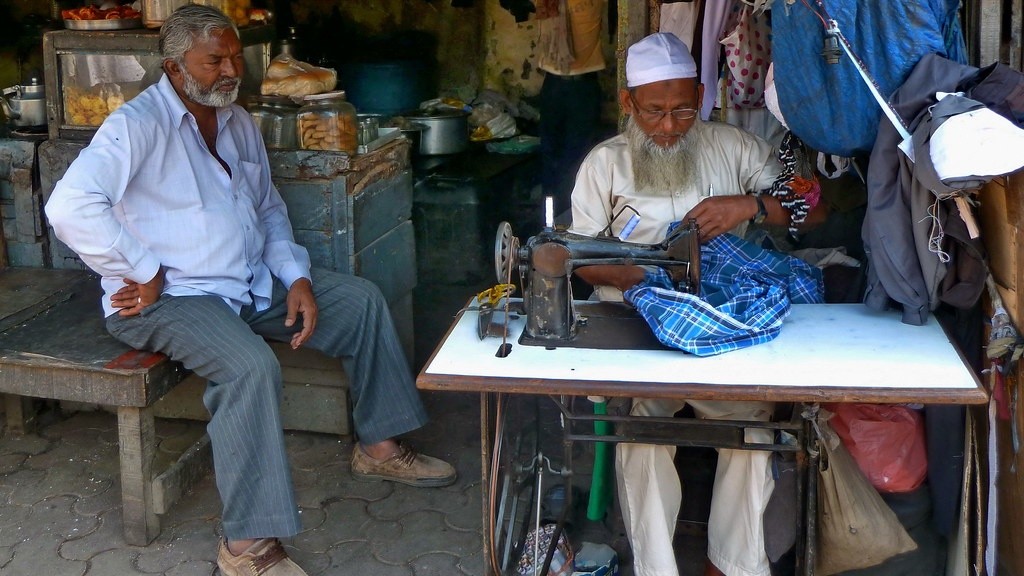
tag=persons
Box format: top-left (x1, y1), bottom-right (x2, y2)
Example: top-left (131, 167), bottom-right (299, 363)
top-left (566, 31), bottom-right (825, 576)
top-left (44, 4), bottom-right (456, 576)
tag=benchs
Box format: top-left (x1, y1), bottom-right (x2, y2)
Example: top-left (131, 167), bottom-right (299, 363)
top-left (0, 265), bottom-right (214, 548)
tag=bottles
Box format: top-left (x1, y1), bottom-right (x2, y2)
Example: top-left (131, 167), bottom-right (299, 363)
top-left (356, 113), bottom-right (382, 152)
top-left (296, 90), bottom-right (360, 152)
top-left (247, 95), bottom-right (299, 150)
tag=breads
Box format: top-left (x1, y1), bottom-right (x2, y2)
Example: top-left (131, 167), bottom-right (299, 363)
top-left (67, 94), bottom-right (131, 126)
top-left (62, 4), bottom-right (142, 19)
top-left (260, 59), bottom-right (337, 98)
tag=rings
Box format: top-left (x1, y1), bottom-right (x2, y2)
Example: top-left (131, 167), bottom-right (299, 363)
top-left (138, 296), bottom-right (141, 303)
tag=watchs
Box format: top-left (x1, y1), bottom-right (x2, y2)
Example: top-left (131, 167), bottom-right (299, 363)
top-left (751, 196), bottom-right (768, 224)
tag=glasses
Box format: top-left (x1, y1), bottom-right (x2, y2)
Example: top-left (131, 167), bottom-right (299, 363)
top-left (631, 97), bottom-right (698, 122)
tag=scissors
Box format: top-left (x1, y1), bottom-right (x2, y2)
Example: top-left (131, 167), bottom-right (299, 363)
top-left (478, 283), bottom-right (515, 341)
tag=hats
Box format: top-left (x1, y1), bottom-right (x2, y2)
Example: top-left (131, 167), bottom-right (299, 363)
top-left (625, 32), bottom-right (697, 89)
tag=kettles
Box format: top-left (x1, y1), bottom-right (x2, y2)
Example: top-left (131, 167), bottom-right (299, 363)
top-left (0, 76), bottom-right (48, 126)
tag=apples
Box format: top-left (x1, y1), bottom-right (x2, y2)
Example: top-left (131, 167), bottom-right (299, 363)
top-left (247, 7), bottom-right (271, 25)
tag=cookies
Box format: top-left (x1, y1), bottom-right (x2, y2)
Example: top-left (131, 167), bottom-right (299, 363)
top-left (295, 110), bottom-right (358, 152)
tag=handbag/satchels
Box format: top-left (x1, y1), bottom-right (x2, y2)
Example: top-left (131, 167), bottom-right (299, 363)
top-left (823, 402), bottom-right (928, 493)
top-left (813, 419), bottom-right (918, 576)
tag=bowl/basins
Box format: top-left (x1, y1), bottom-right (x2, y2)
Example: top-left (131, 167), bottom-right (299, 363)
top-left (380, 122), bottom-right (431, 161)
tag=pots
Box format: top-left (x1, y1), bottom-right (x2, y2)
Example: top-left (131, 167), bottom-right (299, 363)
top-left (403, 111), bottom-right (473, 156)
top-left (140, 0), bottom-right (251, 29)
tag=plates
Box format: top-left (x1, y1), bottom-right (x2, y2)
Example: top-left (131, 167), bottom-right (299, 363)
top-left (64, 18), bottom-right (142, 30)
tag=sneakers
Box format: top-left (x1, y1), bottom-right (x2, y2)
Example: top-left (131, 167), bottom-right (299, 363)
top-left (215, 536), bottom-right (308, 576)
top-left (351, 440), bottom-right (457, 487)
